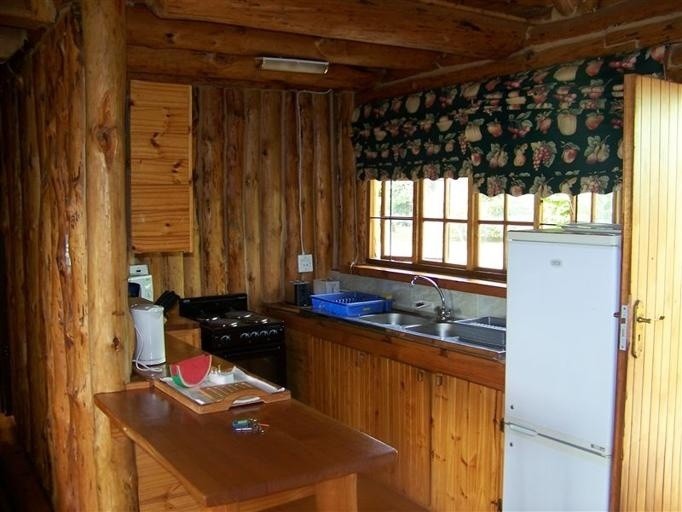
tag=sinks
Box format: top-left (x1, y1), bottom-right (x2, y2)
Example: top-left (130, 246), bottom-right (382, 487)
top-left (359, 313), bottom-right (426, 326)
top-left (405, 324), bottom-right (459, 337)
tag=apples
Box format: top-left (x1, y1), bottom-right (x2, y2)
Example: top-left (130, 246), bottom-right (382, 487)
top-left (586, 114), bottom-right (603, 129)
top-left (558, 113), bottom-right (576, 134)
top-left (466, 122), bottom-right (482, 141)
top-left (488, 124), bottom-right (502, 137)
top-left (563, 145), bottom-right (576, 164)
top-left (537, 117), bottom-right (551, 130)
top-left (406, 97), bottom-right (420, 113)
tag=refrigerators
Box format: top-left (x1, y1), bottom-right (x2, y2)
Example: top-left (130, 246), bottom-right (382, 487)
top-left (501, 221), bottom-right (625, 512)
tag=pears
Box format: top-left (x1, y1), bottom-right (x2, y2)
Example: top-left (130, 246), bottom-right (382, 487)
top-left (514, 149), bottom-right (525, 166)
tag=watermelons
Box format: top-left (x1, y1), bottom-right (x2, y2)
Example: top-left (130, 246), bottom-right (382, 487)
top-left (170, 355), bottom-right (212, 388)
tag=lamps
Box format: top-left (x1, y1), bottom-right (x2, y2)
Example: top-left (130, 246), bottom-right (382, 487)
top-left (253, 55), bottom-right (331, 77)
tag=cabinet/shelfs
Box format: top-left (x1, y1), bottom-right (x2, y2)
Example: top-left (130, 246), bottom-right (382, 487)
top-left (259, 301), bottom-right (505, 512)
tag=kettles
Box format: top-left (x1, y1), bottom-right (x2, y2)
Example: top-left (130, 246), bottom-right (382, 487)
top-left (130, 303), bottom-right (166, 366)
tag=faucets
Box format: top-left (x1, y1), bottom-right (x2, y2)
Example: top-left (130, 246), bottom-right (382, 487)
top-left (410, 275), bottom-right (446, 320)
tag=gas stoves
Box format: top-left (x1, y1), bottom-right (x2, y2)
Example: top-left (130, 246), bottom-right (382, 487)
top-left (179, 292), bottom-right (285, 347)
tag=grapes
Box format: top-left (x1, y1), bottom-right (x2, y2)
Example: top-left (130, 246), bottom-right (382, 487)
top-left (533, 143), bottom-right (552, 168)
top-left (486, 178), bottom-right (501, 196)
top-left (425, 163), bottom-right (437, 180)
